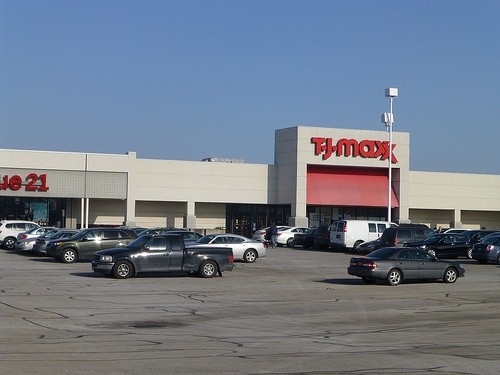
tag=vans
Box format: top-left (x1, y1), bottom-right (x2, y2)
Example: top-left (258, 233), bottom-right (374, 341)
top-left (330, 219), bottom-right (400, 252)
top-left (382, 223), bottom-right (435, 247)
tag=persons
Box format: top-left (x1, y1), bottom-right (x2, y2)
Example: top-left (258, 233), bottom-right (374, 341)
top-left (269, 221), bottom-right (277, 248)
top-left (433, 226), bottom-right (446, 233)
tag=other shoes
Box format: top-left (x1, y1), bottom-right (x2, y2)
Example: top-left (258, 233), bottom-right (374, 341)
top-left (275, 244), bottom-right (277, 248)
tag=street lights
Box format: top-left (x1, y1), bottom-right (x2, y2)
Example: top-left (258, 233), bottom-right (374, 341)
top-left (382, 87), bottom-right (399, 222)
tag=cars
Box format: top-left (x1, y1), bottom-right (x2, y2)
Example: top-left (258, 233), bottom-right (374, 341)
top-left (15, 227), bottom-right (58, 255)
top-left (132, 228), bottom-right (216, 244)
top-left (425, 228), bottom-right (500, 242)
top-left (254, 225), bottom-right (312, 247)
top-left (348, 246), bottom-right (465, 283)
top-left (188, 233), bottom-right (267, 262)
top-left (403, 233), bottom-right (479, 259)
top-left (471, 235), bottom-right (500, 264)
top-left (360, 236), bottom-right (381, 253)
top-left (294, 227), bottom-right (317, 248)
top-left (36, 228), bottom-right (93, 256)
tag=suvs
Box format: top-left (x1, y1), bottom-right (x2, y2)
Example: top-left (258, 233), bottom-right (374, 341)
top-left (47, 228), bottom-right (141, 264)
top-left (314, 225), bottom-right (336, 249)
top-left (0, 219), bottom-right (46, 251)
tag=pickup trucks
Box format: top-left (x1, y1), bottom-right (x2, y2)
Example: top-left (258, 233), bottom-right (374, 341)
top-left (91, 233), bottom-right (236, 279)
